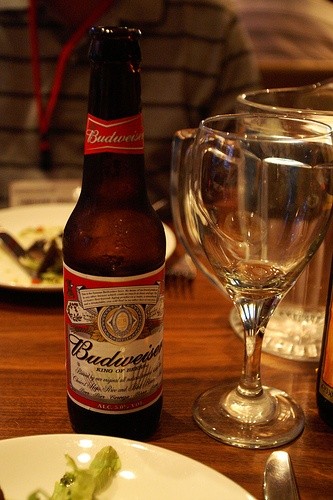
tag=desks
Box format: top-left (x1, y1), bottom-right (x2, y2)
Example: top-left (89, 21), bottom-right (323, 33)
top-left (0, 182), bottom-right (333, 500)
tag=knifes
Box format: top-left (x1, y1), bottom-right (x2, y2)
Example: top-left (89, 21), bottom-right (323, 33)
top-left (263, 450), bottom-right (300, 500)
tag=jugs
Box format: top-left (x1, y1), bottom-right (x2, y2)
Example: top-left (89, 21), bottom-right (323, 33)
top-left (169, 78), bottom-right (333, 362)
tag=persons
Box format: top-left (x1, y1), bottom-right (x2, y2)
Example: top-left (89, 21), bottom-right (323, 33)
top-left (0, 0), bottom-right (258, 206)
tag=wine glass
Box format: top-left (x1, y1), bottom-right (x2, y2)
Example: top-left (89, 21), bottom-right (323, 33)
top-left (179, 111), bottom-right (333, 450)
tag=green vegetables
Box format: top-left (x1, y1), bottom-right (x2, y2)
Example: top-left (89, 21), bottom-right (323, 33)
top-left (18, 257), bottom-right (62, 281)
top-left (26, 445), bottom-right (121, 500)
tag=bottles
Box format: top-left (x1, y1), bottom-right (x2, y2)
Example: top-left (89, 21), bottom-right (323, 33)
top-left (315, 267), bottom-right (333, 432)
top-left (61, 24), bottom-right (166, 438)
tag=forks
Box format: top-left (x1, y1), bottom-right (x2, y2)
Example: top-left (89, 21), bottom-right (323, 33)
top-left (166, 251), bottom-right (197, 281)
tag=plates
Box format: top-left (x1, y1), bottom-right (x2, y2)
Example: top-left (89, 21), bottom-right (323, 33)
top-left (0, 434), bottom-right (258, 500)
top-left (0, 203), bottom-right (176, 291)
